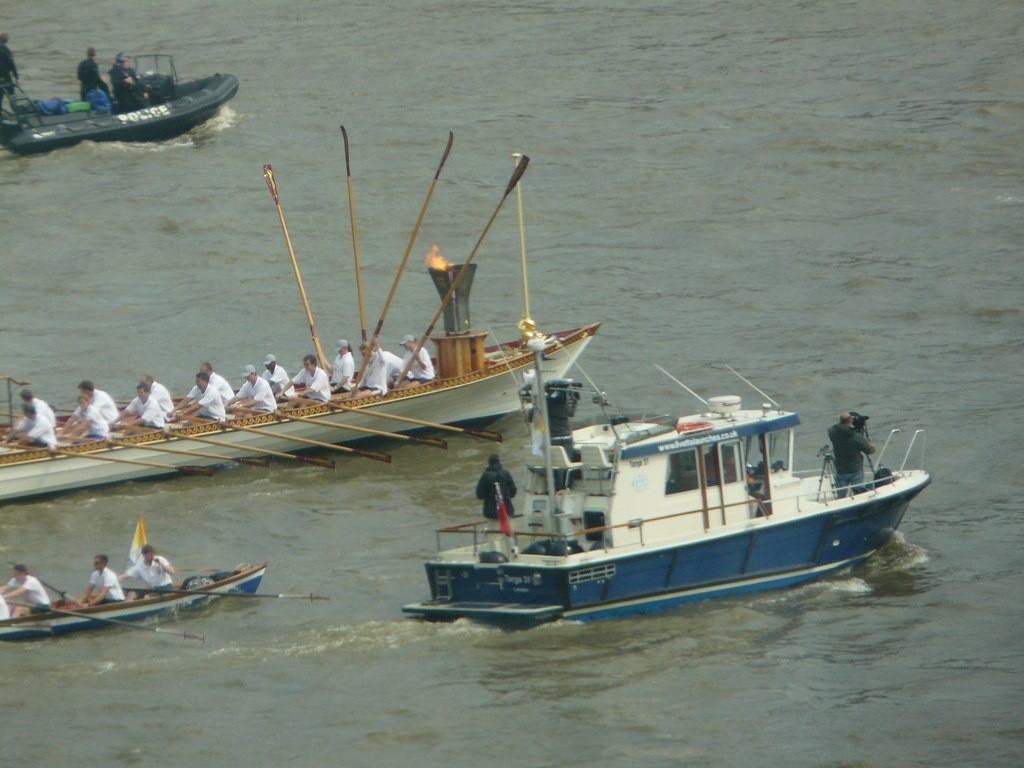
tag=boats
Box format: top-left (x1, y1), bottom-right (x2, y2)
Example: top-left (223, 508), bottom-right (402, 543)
top-left (0, 564), bottom-right (269, 639)
top-left (0, 262), bottom-right (605, 501)
top-left (401, 152), bottom-right (934, 623)
top-left (1, 54), bottom-right (239, 155)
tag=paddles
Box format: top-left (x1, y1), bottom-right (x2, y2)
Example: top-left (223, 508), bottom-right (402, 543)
top-left (108, 423), bottom-right (336, 469)
top-left (121, 586), bottom-right (331, 602)
top-left (280, 396), bottom-right (503, 442)
top-left (57, 436), bottom-right (270, 469)
top-left (0, 443), bottom-right (214, 477)
top-left (5, 599), bottom-right (205, 641)
top-left (0, 412), bottom-right (67, 426)
top-left (114, 396), bottom-right (182, 404)
top-left (394, 153), bottom-right (530, 390)
top-left (350, 128), bottom-right (455, 398)
top-left (7, 561), bottom-right (81, 606)
top-left (226, 405), bottom-right (448, 450)
top-left (167, 413), bottom-right (392, 463)
top-left (339, 125), bottom-right (367, 358)
top-left (50, 403), bottom-right (76, 414)
top-left (262, 163), bottom-right (328, 372)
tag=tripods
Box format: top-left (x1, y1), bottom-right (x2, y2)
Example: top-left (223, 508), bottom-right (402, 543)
top-left (816, 454), bottom-right (844, 502)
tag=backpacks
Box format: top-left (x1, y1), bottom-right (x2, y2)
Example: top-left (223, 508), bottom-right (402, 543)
top-left (874, 464), bottom-right (895, 488)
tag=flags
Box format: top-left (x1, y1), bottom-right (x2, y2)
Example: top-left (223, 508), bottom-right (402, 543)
top-left (495, 485), bottom-right (514, 538)
top-left (126, 519), bottom-right (147, 579)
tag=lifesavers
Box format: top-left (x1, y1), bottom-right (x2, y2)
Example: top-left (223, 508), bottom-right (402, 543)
top-left (676, 422), bottom-right (713, 434)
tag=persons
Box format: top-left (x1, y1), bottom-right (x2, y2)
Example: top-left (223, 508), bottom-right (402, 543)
top-left (526, 381), bottom-right (579, 463)
top-left (77, 46), bottom-right (107, 102)
top-left (476, 453), bottom-right (518, 560)
top-left (62, 380), bottom-right (119, 447)
top-left (327, 339), bottom-right (354, 395)
top-left (115, 544), bottom-right (175, 603)
top-left (80, 554), bottom-right (126, 608)
top-left (0, 32), bottom-right (18, 111)
top-left (167, 362), bottom-right (234, 428)
top-left (349, 334), bottom-right (435, 397)
top-left (0, 563), bottom-right (52, 621)
top-left (828, 412), bottom-right (870, 499)
top-left (276, 354), bottom-right (331, 412)
top-left (3, 388), bottom-right (57, 452)
top-left (108, 51), bottom-right (152, 111)
top-left (708, 447), bottom-right (759, 518)
top-left (226, 353), bottom-right (296, 420)
top-left (111, 373), bottom-right (175, 437)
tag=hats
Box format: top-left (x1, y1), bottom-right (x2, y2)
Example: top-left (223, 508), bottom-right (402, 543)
top-left (262, 354), bottom-right (276, 364)
top-left (334, 339), bottom-right (349, 351)
top-left (240, 365), bottom-right (255, 376)
top-left (399, 335), bottom-right (415, 344)
